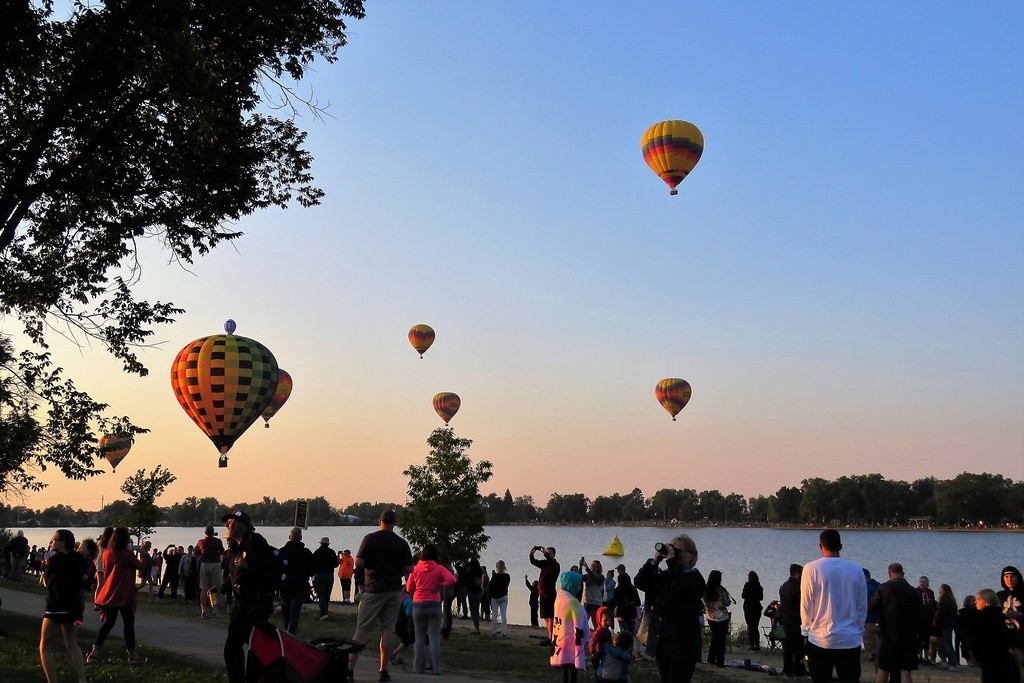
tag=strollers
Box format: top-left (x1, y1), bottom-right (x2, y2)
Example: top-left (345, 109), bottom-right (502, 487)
top-left (234, 595), bottom-right (366, 682)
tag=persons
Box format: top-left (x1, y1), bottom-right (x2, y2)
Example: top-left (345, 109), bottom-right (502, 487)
top-left (742, 571), bottom-right (763, 653)
top-left (529, 546), bottom-right (560, 647)
top-left (764, 563), bottom-right (809, 676)
top-left (391, 544), bottom-right (510, 675)
top-left (703, 570), bottom-right (731, 668)
top-left (549, 560), bottom-right (641, 683)
top-left (525, 578), bottom-right (539, 627)
top-left (800, 528), bottom-right (868, 683)
top-left (347, 510), bottom-right (414, 683)
top-left (206, 510), bottom-right (284, 683)
top-left (864, 563), bottom-right (1024, 683)
top-left (6, 526), bottom-right (231, 663)
top-left (39, 530), bottom-right (88, 683)
top-left (270, 528), bottom-right (364, 635)
top-left (634, 534), bottom-right (706, 683)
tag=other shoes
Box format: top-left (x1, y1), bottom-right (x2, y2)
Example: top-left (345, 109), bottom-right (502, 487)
top-left (471, 632), bottom-right (481, 635)
top-left (750, 647), bottom-right (761, 653)
top-left (502, 634), bottom-right (507, 637)
top-left (156, 596), bottom-right (161, 599)
top-left (173, 597), bottom-right (178, 600)
top-left (319, 614), bottom-right (329, 621)
top-left (127, 656), bottom-right (149, 665)
top-left (492, 634), bottom-right (496, 637)
top-left (781, 673), bottom-right (811, 681)
top-left (213, 613), bottom-right (224, 619)
top-left (706, 661), bottom-right (725, 669)
top-left (346, 670), bottom-right (354, 682)
top-left (86, 654), bottom-right (103, 664)
top-left (540, 639), bottom-right (552, 646)
top-left (378, 670), bottom-right (390, 682)
top-left (919, 657), bottom-right (961, 672)
top-left (458, 616), bottom-right (468, 620)
top-left (188, 600), bottom-right (195, 605)
top-left (200, 613), bottom-right (211, 619)
top-left (482, 618), bottom-right (491, 621)
top-left (0, 629), bottom-right (9, 639)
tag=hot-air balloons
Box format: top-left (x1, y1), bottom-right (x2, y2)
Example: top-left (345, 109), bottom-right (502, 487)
top-left (170, 334), bottom-right (279, 468)
top-left (433, 392), bottom-right (461, 426)
top-left (408, 324), bottom-right (435, 359)
top-left (99, 434), bottom-right (131, 473)
top-left (641, 120), bottom-right (705, 195)
top-left (224, 319), bottom-right (236, 335)
top-left (261, 369), bottom-right (293, 427)
top-left (654, 378), bottom-right (691, 421)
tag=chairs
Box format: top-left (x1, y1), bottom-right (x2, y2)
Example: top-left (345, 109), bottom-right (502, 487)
top-left (703, 612), bottom-right (734, 655)
top-left (762, 626), bottom-right (784, 656)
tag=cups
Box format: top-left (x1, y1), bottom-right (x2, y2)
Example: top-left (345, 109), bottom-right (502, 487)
top-left (209, 587), bottom-right (218, 606)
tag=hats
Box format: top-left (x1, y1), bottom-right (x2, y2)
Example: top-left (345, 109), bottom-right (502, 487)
top-left (354, 589), bottom-right (362, 603)
top-left (221, 509), bottom-right (254, 530)
top-left (790, 563), bottom-right (804, 576)
top-left (319, 537), bottom-right (330, 545)
top-left (615, 564), bottom-right (625, 569)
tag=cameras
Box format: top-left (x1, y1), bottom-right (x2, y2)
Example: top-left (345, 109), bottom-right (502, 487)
top-left (655, 543), bottom-right (679, 557)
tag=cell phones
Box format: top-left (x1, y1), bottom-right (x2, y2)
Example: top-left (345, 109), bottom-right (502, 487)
top-left (133, 545), bottom-right (140, 550)
top-left (339, 551), bottom-right (342, 554)
top-left (170, 545), bottom-right (175, 546)
top-left (535, 546), bottom-right (541, 550)
top-left (582, 557), bottom-right (584, 563)
